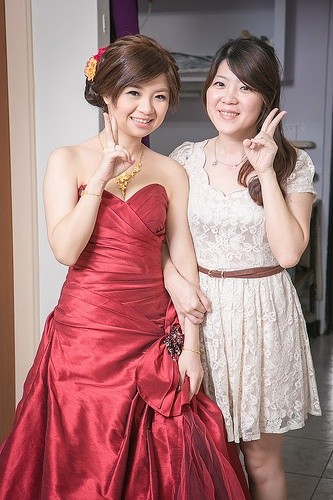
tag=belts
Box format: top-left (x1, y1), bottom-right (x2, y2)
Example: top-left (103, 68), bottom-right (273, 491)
top-left (197, 264), bottom-right (286, 279)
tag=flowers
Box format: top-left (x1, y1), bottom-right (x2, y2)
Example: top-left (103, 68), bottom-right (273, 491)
top-left (84, 47), bottom-right (106, 82)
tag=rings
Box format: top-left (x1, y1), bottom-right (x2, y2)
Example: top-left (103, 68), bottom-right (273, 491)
top-left (113, 143), bottom-right (118, 150)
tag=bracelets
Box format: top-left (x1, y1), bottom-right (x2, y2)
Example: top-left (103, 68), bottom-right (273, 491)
top-left (181, 347), bottom-right (202, 356)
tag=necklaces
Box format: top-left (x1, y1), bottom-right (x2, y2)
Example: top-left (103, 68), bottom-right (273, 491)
top-left (98, 130), bottom-right (145, 201)
top-left (211, 136), bottom-right (246, 168)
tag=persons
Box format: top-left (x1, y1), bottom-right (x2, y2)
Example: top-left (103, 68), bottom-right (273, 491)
top-left (157, 36), bottom-right (324, 500)
top-left (0, 33), bottom-right (251, 500)
